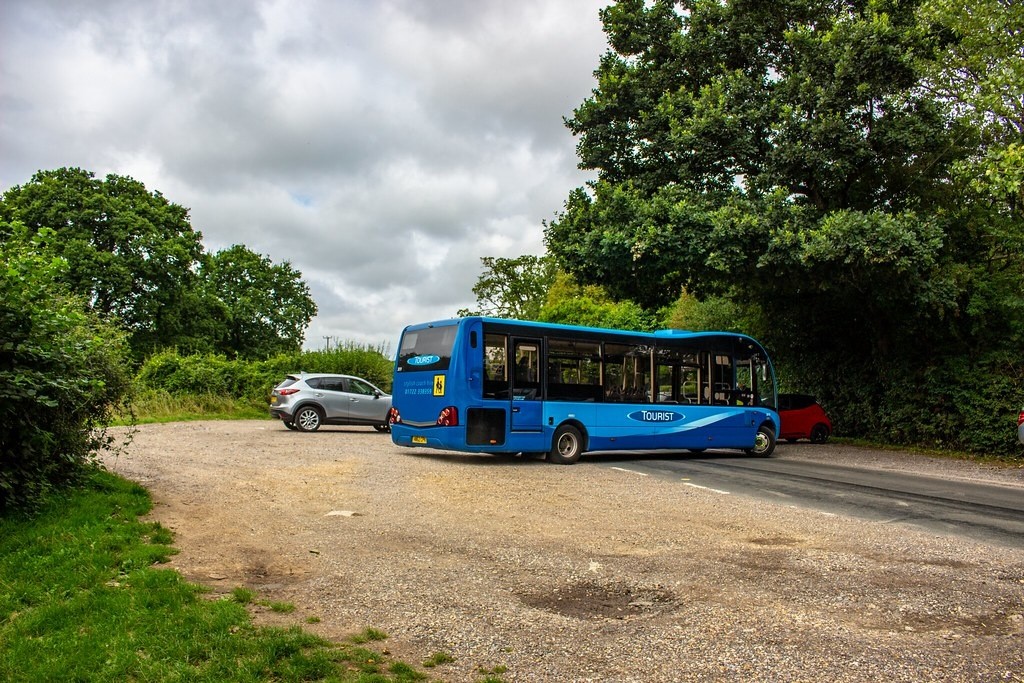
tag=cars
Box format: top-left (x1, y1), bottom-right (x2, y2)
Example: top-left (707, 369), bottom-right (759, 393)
top-left (752, 393), bottom-right (832, 444)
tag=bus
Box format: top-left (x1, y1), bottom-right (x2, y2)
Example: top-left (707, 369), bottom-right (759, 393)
top-left (389, 316), bottom-right (781, 465)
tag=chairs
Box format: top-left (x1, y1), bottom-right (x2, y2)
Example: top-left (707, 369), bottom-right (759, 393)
top-left (517, 356), bottom-right (532, 382)
top-left (494, 365), bottom-right (505, 381)
top-left (550, 361), bottom-right (564, 384)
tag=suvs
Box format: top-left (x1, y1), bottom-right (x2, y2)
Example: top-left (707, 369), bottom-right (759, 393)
top-left (269, 371), bottom-right (394, 433)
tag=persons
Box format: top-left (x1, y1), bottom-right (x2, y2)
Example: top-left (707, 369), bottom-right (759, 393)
top-left (519, 356), bottom-right (529, 368)
